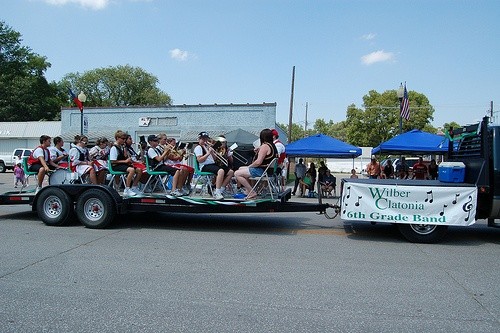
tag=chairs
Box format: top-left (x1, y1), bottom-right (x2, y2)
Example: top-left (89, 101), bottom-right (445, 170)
top-left (20, 157), bottom-right (37, 194)
top-left (190, 153), bottom-right (213, 198)
top-left (244, 158), bottom-right (279, 200)
top-left (108, 155), bottom-right (126, 188)
top-left (322, 177), bottom-right (336, 198)
top-left (142, 155), bottom-right (168, 194)
top-left (68, 155), bottom-right (87, 184)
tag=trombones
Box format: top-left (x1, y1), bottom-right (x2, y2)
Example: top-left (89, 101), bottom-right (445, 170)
top-left (226, 146), bottom-right (248, 164)
top-left (204, 141), bottom-right (228, 167)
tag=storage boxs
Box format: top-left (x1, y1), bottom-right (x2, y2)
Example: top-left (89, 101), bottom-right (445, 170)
top-left (438, 162), bottom-right (466, 183)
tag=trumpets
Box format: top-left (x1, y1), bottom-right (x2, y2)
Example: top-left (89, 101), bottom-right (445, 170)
top-left (157, 144), bottom-right (181, 158)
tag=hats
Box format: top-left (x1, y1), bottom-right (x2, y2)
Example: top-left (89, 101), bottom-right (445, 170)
top-left (148, 135), bottom-right (161, 142)
top-left (270, 129), bottom-right (278, 135)
top-left (214, 137), bottom-right (226, 142)
top-left (198, 132), bottom-right (212, 140)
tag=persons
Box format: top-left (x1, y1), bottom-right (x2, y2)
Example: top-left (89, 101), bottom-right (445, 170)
top-left (321, 169), bottom-right (335, 199)
top-left (291, 158), bottom-right (307, 196)
top-left (428, 159), bottom-right (439, 181)
top-left (69, 134), bottom-right (105, 186)
top-left (404, 168), bottom-right (417, 180)
top-left (89, 136), bottom-right (120, 192)
top-left (318, 161), bottom-right (328, 197)
top-left (14, 163), bottom-right (27, 188)
top-left (350, 169), bottom-right (358, 179)
top-left (383, 160), bottom-right (394, 179)
top-left (413, 157), bottom-right (429, 180)
top-left (27, 134), bottom-right (72, 195)
top-left (299, 162), bottom-right (316, 198)
top-left (109, 128), bottom-right (289, 200)
top-left (367, 155), bottom-right (382, 179)
top-left (395, 156), bottom-right (410, 179)
top-left (16, 153), bottom-right (30, 186)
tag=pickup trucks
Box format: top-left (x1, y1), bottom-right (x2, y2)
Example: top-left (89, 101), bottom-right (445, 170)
top-left (0, 148), bottom-right (36, 173)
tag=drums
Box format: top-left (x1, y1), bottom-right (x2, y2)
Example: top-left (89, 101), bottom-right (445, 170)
top-left (48, 167), bottom-right (74, 185)
top-left (93, 160), bottom-right (104, 172)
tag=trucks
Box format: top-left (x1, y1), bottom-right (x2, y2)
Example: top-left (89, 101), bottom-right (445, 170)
top-left (335, 114), bottom-right (500, 244)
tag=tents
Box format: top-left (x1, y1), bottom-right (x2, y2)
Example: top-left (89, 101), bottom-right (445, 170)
top-left (371, 128), bottom-right (459, 166)
top-left (283, 134), bottom-right (362, 176)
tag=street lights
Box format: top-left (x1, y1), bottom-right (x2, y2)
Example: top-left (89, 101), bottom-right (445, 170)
top-left (77, 91), bottom-right (88, 135)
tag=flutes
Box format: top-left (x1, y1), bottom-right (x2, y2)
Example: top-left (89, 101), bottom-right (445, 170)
top-left (123, 144), bottom-right (132, 161)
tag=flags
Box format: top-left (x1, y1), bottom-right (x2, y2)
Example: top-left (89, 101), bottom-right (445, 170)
top-left (401, 85), bottom-right (411, 121)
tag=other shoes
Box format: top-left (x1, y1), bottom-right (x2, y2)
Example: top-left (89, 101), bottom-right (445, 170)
top-left (291, 192), bottom-right (295, 196)
top-left (14, 185), bottom-right (18, 188)
top-left (182, 185), bottom-right (193, 194)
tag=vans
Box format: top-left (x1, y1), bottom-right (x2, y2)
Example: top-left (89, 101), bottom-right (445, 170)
top-left (379, 157), bottom-right (420, 174)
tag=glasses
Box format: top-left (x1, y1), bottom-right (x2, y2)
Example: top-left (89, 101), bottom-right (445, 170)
top-left (82, 141), bottom-right (87, 144)
top-left (119, 137), bottom-right (127, 140)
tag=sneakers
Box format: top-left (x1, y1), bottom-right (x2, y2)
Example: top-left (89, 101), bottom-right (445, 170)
top-left (34, 186), bottom-right (42, 195)
top-left (221, 190), bottom-right (231, 196)
top-left (215, 191), bottom-right (224, 200)
top-left (170, 188), bottom-right (189, 196)
top-left (131, 186), bottom-right (144, 194)
top-left (123, 187), bottom-right (137, 196)
